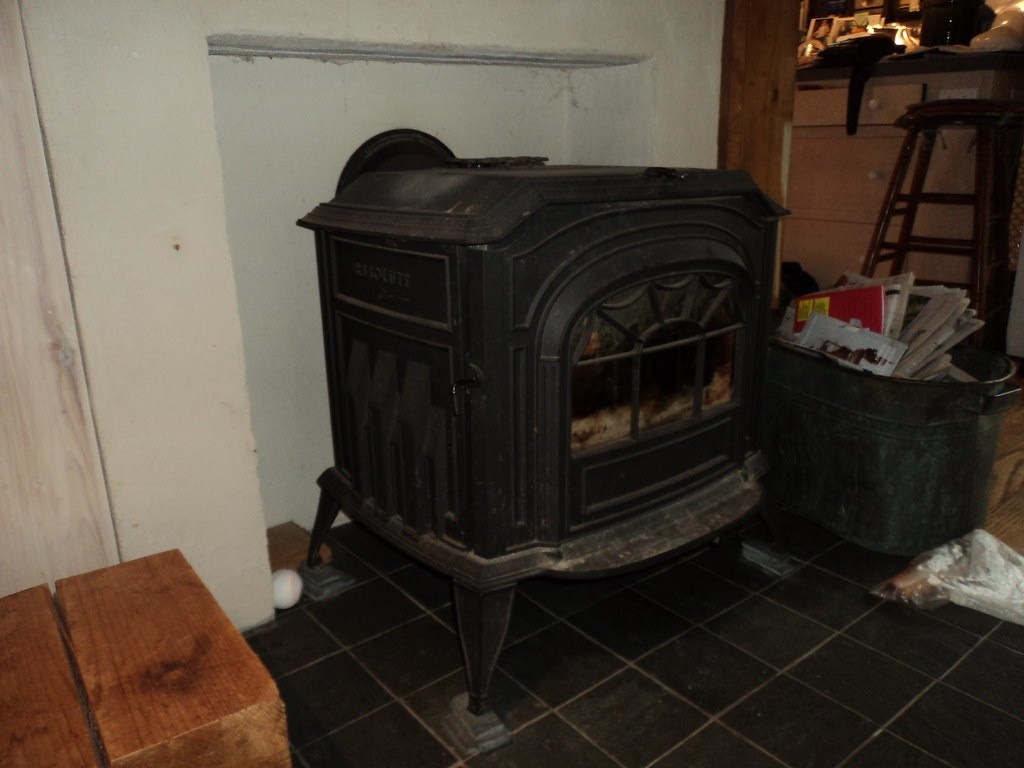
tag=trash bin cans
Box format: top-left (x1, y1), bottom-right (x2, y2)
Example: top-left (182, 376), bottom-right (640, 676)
top-left (767, 329), bottom-right (1023, 557)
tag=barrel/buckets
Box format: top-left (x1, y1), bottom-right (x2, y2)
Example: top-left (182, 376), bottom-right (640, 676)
top-left (774, 338), bottom-right (1024, 556)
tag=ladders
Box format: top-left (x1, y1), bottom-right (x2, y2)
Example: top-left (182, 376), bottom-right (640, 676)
top-left (860, 95), bottom-right (990, 290)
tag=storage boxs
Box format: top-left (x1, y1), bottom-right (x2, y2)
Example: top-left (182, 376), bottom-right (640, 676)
top-left (0, 548), bottom-right (293, 768)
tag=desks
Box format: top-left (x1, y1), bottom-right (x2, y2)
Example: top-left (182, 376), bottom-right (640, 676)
top-left (795, 50), bottom-right (1024, 134)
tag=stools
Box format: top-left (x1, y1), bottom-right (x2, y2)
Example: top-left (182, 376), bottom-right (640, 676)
top-left (864, 98), bottom-right (1024, 344)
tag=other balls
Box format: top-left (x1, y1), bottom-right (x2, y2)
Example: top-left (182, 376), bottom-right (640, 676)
top-left (272, 569), bottom-right (303, 609)
